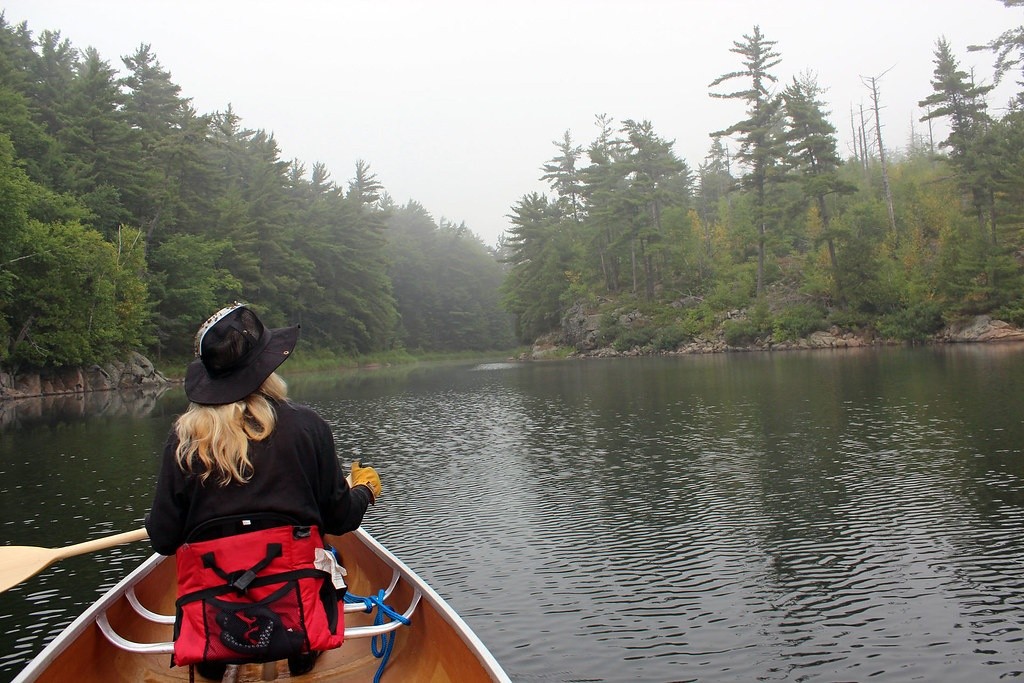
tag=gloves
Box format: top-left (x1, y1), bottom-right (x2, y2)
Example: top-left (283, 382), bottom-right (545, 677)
top-left (350, 461), bottom-right (381, 505)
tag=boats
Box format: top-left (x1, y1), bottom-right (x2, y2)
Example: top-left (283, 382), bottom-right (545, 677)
top-left (4, 510), bottom-right (516, 683)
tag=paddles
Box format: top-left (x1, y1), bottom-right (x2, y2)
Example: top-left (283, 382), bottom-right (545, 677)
top-left (0, 473), bottom-right (354, 591)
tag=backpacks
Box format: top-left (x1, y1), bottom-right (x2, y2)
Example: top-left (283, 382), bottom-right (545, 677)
top-left (171, 514), bottom-right (344, 667)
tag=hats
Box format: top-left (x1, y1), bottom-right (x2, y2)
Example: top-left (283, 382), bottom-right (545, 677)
top-left (185, 302), bottom-right (301, 406)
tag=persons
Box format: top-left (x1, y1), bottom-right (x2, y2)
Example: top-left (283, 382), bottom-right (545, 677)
top-left (146, 304), bottom-right (383, 680)
